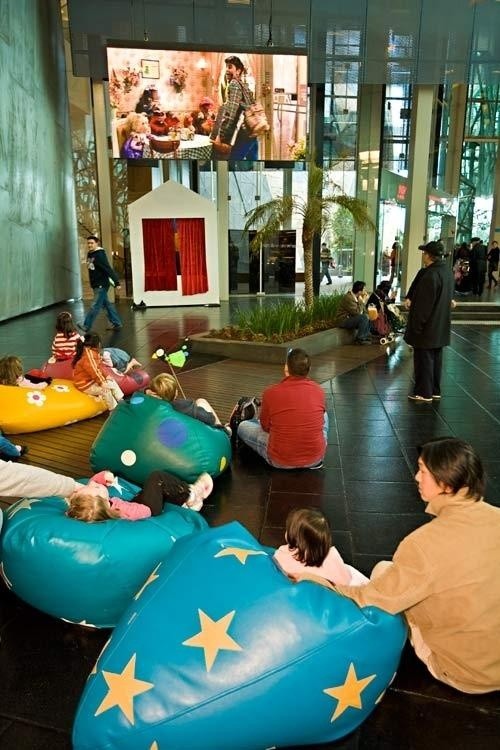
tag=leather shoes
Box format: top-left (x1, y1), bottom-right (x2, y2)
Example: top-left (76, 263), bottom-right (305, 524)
top-left (18, 444), bottom-right (30, 455)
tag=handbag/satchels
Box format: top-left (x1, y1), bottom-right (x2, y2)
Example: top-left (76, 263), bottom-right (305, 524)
top-left (243, 100), bottom-right (271, 136)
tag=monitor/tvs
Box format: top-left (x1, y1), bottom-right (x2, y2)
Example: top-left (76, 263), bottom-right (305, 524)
top-left (105, 40), bottom-right (308, 163)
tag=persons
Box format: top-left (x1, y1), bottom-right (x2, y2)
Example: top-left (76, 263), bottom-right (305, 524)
top-left (291, 436), bottom-right (499, 695)
top-left (320, 243), bottom-right (333, 285)
top-left (236, 347), bottom-right (329, 470)
top-left (77, 236), bottom-right (123, 332)
top-left (144, 373), bottom-right (221, 426)
top-left (404, 241), bottom-right (456, 401)
top-left (0, 438), bottom-right (213, 522)
top-left (1, 312), bottom-right (144, 414)
top-left (217, 56), bottom-right (258, 161)
top-left (269, 506), bottom-right (370, 587)
top-left (338, 236), bottom-right (500, 346)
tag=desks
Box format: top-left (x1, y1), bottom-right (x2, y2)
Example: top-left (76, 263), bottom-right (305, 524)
top-left (154, 134), bottom-right (213, 159)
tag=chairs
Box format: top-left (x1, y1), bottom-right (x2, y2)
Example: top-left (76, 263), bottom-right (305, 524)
top-left (112, 118), bottom-right (129, 158)
top-left (147, 135), bottom-right (180, 159)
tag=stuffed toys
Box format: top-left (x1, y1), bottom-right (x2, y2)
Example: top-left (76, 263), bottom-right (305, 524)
top-left (122, 89), bottom-right (216, 159)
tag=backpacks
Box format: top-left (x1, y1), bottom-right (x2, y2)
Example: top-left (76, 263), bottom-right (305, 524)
top-left (228, 395), bottom-right (259, 435)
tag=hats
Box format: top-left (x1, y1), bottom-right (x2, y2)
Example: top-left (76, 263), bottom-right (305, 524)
top-left (419, 241), bottom-right (445, 255)
top-left (224, 57), bottom-right (243, 69)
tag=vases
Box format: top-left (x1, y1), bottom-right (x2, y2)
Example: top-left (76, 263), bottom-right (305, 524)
top-left (295, 159), bottom-right (305, 161)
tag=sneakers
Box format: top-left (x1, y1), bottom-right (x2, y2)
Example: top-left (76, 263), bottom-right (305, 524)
top-left (77, 324), bottom-right (88, 332)
top-left (107, 322), bottom-right (122, 330)
top-left (407, 392), bottom-right (441, 402)
top-left (184, 472), bottom-right (213, 511)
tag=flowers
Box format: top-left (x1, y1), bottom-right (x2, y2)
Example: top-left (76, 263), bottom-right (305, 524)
top-left (109, 69), bottom-right (121, 108)
top-left (287, 138), bottom-right (306, 160)
top-left (172, 65), bottom-right (188, 93)
top-left (122, 67), bottom-right (141, 87)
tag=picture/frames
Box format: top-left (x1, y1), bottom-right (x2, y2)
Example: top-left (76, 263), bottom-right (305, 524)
top-left (141, 58), bottom-right (160, 79)
